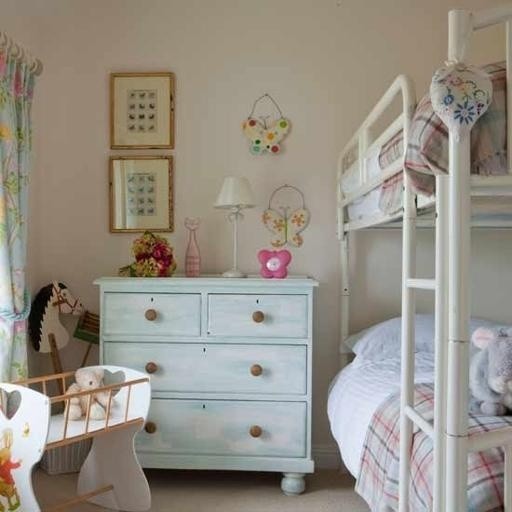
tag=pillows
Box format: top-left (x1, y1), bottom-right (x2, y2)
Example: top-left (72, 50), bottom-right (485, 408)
top-left (344, 314), bottom-right (504, 358)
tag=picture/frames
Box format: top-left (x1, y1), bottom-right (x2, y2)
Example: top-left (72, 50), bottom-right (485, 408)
top-left (108, 155), bottom-right (175, 232)
top-left (109, 71), bottom-right (175, 149)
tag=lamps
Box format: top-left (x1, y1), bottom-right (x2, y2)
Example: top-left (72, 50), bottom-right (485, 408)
top-left (212, 175), bottom-right (255, 277)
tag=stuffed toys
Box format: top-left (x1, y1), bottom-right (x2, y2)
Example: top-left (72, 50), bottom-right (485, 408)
top-left (468, 327), bottom-right (512, 416)
top-left (64, 367), bottom-right (114, 420)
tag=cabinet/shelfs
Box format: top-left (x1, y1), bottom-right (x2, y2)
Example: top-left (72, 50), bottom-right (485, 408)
top-left (1, 367), bottom-right (151, 511)
top-left (92, 275), bottom-right (318, 495)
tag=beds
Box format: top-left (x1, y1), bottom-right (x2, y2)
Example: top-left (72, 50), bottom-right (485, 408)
top-left (336, 10), bottom-right (511, 512)
